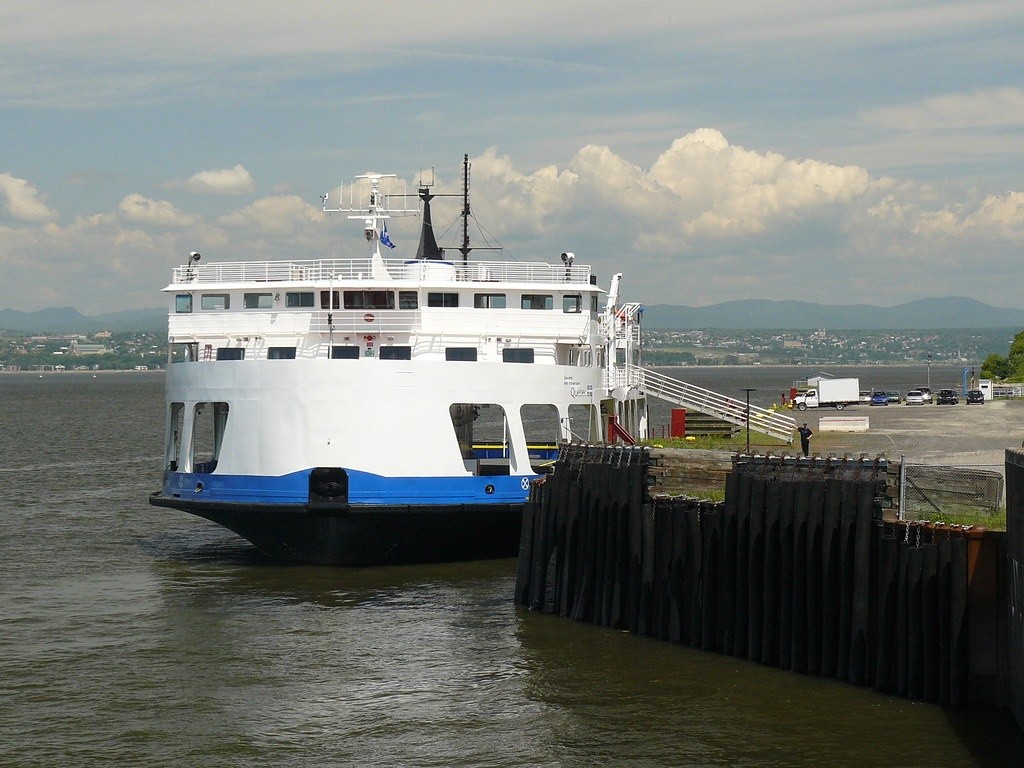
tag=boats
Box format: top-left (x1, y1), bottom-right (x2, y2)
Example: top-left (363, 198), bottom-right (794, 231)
top-left (145, 148), bottom-right (649, 572)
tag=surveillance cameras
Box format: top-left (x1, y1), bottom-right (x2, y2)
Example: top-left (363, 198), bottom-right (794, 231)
top-left (559, 251), bottom-right (575, 262)
top-left (188, 250), bottom-right (201, 261)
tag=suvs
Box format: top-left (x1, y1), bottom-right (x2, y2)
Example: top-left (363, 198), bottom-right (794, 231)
top-left (885, 389), bottom-right (901, 404)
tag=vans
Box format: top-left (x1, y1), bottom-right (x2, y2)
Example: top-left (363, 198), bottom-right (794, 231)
top-left (915, 386), bottom-right (934, 404)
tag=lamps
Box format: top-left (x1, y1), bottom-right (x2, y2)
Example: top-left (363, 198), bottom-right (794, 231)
top-left (274, 291), bottom-right (280, 302)
top-left (186, 251), bottom-right (202, 280)
top-left (560, 252), bottom-right (575, 280)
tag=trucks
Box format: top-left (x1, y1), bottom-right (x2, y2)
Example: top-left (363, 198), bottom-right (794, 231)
top-left (794, 377), bottom-right (860, 411)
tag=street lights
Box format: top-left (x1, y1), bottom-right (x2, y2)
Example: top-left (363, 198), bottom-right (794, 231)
top-left (927, 351), bottom-right (932, 387)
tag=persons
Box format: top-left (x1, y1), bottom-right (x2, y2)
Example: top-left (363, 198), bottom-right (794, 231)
top-left (792, 422), bottom-right (813, 457)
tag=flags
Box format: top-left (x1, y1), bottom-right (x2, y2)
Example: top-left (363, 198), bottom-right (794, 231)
top-left (380, 220), bottom-right (396, 250)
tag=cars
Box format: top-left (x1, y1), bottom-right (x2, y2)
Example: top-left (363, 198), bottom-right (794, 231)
top-left (859, 391), bottom-right (872, 404)
top-left (965, 389), bottom-right (986, 404)
top-left (937, 389), bottom-right (959, 405)
top-left (868, 390), bottom-right (889, 406)
top-left (904, 391), bottom-right (924, 406)
